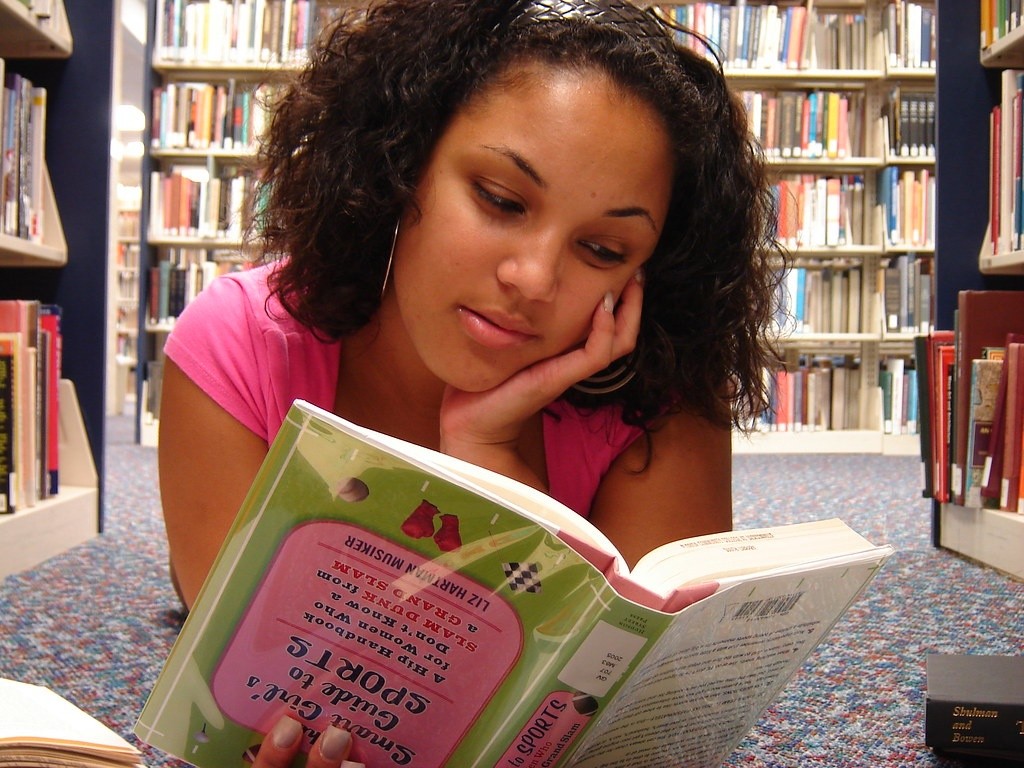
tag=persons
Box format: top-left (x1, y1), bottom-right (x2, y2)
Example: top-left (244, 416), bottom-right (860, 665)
top-left (158, 1), bottom-right (800, 768)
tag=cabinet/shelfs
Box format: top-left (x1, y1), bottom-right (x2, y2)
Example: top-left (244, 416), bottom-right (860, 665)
top-left (931, 0), bottom-right (1024, 581)
top-left (118, 209), bottom-right (140, 418)
top-left (0, 1), bottom-right (120, 581)
top-left (135, 0), bottom-right (936, 456)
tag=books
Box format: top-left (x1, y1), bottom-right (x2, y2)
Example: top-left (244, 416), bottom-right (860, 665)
top-left (142, 2), bottom-right (328, 415)
top-left (0, 674), bottom-right (149, 768)
top-left (135, 399), bottom-right (897, 766)
top-left (916, 2), bottom-right (1023, 512)
top-left (668, 0), bottom-right (937, 435)
top-left (925, 652), bottom-right (1024, 757)
top-left (0, 0), bottom-right (62, 522)
